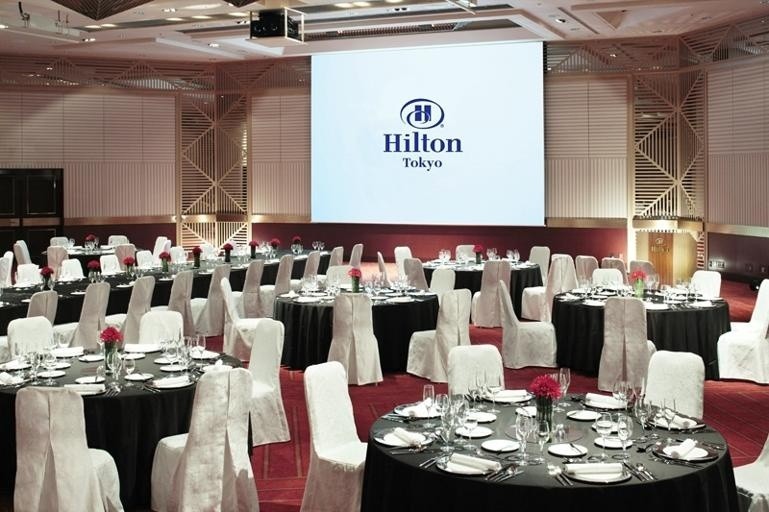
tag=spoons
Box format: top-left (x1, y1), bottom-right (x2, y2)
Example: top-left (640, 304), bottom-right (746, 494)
top-left (547, 463), bottom-right (575, 487)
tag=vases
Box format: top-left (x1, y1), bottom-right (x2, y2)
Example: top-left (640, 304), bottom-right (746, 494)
top-left (535, 393), bottom-right (554, 443)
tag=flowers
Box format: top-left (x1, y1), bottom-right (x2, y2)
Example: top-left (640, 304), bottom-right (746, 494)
top-left (528, 373), bottom-right (560, 398)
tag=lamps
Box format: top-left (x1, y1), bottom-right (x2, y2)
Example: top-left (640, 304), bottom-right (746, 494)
top-left (0, 2), bottom-right (81, 44)
top-left (156, 36), bottom-right (245, 60)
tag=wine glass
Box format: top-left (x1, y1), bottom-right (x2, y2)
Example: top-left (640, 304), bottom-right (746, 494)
top-left (515, 415), bottom-right (550, 464)
top-left (178, 334), bottom-right (209, 374)
top-left (422, 368), bottom-right (502, 428)
top-left (43, 331), bottom-right (70, 367)
top-left (557, 368), bottom-right (571, 408)
top-left (596, 376), bottom-right (677, 420)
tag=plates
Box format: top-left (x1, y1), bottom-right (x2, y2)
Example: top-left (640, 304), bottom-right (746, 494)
top-left (1, 343), bottom-right (234, 395)
top-left (548, 395), bottom-right (708, 483)
top-left (555, 288), bottom-right (724, 310)
top-left (278, 283), bottom-right (436, 303)
top-left (372, 389), bottom-right (533, 476)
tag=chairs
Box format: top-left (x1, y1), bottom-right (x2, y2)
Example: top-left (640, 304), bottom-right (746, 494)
top-left (644, 350), bottom-right (707, 426)
top-left (300, 362), bottom-right (369, 512)
top-left (715, 277), bottom-right (769, 386)
top-left (13, 388), bottom-right (129, 512)
top-left (730, 433), bottom-right (769, 511)
top-left (1, 236), bottom-right (731, 378)
top-left (242, 316), bottom-right (294, 449)
top-left (445, 342), bottom-right (507, 397)
top-left (594, 295), bottom-right (658, 395)
top-left (406, 288), bottom-right (474, 386)
top-left (326, 293), bottom-right (386, 389)
top-left (150, 365), bottom-right (262, 512)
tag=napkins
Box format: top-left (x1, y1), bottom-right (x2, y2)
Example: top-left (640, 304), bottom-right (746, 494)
top-left (394, 425), bottom-right (419, 448)
top-left (451, 452), bottom-right (500, 471)
top-left (499, 388), bottom-right (530, 399)
top-left (672, 437), bottom-right (699, 457)
top-left (63, 382), bottom-right (108, 392)
top-left (409, 398), bottom-right (434, 418)
top-left (1, 370), bottom-right (24, 387)
top-left (586, 391), bottom-right (626, 407)
top-left (565, 461), bottom-right (623, 477)
top-left (152, 374), bottom-right (189, 386)
